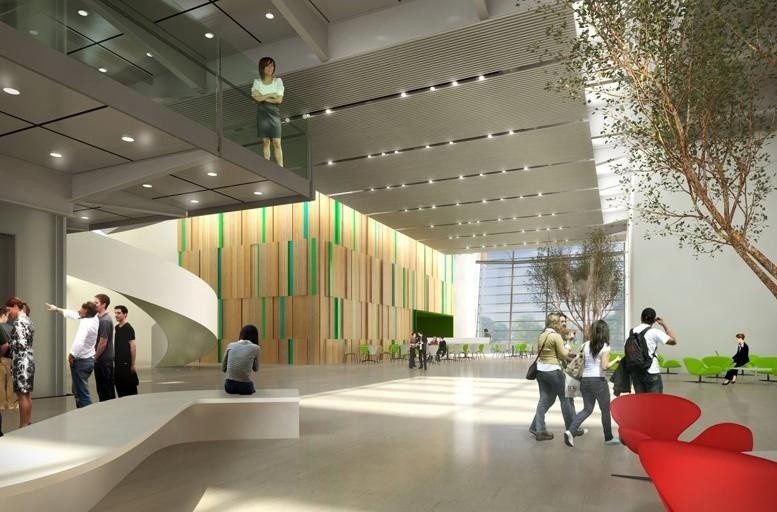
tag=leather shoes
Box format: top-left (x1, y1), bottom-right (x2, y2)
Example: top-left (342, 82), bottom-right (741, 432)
top-left (722, 381), bottom-right (730, 385)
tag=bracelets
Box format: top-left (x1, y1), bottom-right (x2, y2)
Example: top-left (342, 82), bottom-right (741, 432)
top-left (664, 328), bottom-right (672, 335)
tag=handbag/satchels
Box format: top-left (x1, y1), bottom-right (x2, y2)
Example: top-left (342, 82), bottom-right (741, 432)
top-left (527, 363), bottom-right (537, 380)
top-left (565, 352), bottom-right (585, 382)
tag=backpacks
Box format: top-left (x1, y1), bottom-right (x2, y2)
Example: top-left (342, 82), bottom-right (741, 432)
top-left (625, 327), bottom-right (657, 376)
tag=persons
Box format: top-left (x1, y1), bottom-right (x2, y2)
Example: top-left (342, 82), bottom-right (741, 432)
top-left (114, 305), bottom-right (140, 396)
top-left (222, 325), bottom-right (262, 395)
top-left (528, 314), bottom-right (589, 436)
top-left (564, 320), bottom-right (620, 447)
top-left (723, 333), bottom-right (749, 386)
top-left (0, 299), bottom-right (36, 436)
top-left (408, 331), bottom-right (446, 369)
top-left (92, 294), bottom-right (116, 401)
top-left (44, 302), bottom-right (98, 408)
top-left (484, 329), bottom-right (491, 338)
top-left (535, 312), bottom-right (583, 441)
top-left (251, 57), bottom-right (283, 167)
top-left (625, 308), bottom-right (676, 393)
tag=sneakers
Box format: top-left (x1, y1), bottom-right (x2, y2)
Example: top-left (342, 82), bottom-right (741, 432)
top-left (529, 427), bottom-right (553, 440)
top-left (573, 428), bottom-right (583, 436)
top-left (564, 431), bottom-right (575, 447)
top-left (606, 438), bottom-right (620, 444)
